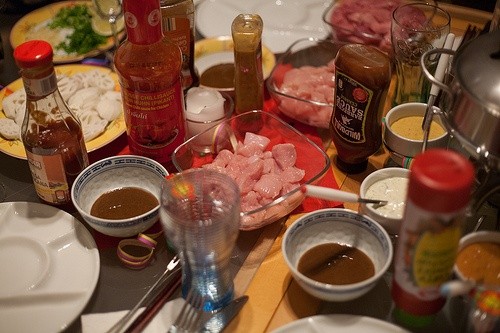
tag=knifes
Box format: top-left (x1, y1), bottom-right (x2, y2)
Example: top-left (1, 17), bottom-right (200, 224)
top-left (200, 295), bottom-right (249, 333)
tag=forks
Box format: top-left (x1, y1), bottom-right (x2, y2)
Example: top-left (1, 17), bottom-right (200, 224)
top-left (167, 289), bottom-right (206, 333)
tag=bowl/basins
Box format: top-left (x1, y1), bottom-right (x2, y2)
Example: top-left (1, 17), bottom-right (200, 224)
top-left (322, 0), bottom-right (438, 65)
top-left (193, 36), bottom-right (276, 97)
top-left (266, 37), bottom-right (394, 129)
top-left (450, 231), bottom-right (500, 325)
top-left (382, 102), bottom-right (449, 158)
top-left (71, 154), bottom-right (170, 237)
top-left (171, 110), bottom-right (411, 302)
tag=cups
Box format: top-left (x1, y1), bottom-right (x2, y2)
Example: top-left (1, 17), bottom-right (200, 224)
top-left (160, 169), bottom-right (241, 311)
top-left (390, 3), bottom-right (452, 108)
top-left (183, 90), bottom-right (234, 154)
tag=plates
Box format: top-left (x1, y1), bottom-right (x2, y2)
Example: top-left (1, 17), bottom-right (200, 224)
top-left (0, 65), bottom-right (126, 160)
top-left (270, 313), bottom-right (412, 333)
top-left (10, 0), bottom-right (126, 64)
top-left (194, 0), bottom-right (336, 54)
top-left (0, 202), bottom-right (100, 333)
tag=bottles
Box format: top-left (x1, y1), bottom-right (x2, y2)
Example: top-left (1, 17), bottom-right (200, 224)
top-left (112, 0), bottom-right (193, 172)
top-left (391, 149), bottom-right (476, 330)
top-left (231, 13), bottom-right (264, 133)
top-left (13, 40), bottom-right (90, 213)
top-left (328, 43), bottom-right (393, 173)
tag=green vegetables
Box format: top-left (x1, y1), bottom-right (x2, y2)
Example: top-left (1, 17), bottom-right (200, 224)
top-left (48, 4), bottom-right (110, 56)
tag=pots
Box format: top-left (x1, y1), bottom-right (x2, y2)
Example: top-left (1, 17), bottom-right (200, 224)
top-left (421, 30), bottom-right (500, 159)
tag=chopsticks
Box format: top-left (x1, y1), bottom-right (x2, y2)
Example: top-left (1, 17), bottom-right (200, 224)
top-left (123, 270), bottom-right (182, 333)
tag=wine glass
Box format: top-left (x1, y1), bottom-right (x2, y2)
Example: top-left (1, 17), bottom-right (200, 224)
top-left (91, 0), bottom-right (124, 50)
